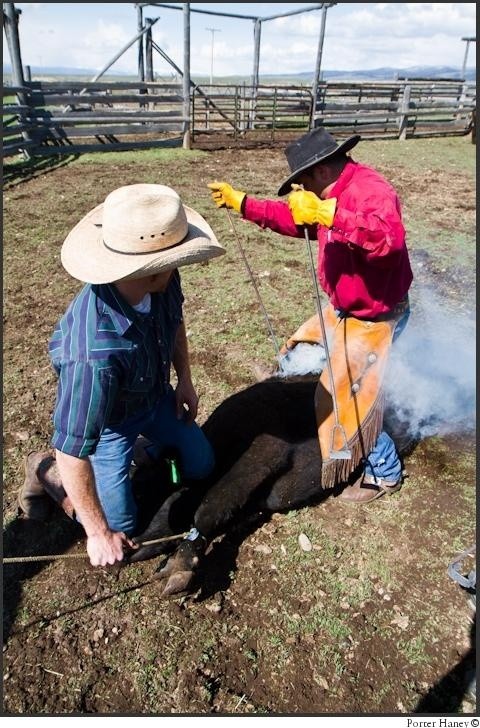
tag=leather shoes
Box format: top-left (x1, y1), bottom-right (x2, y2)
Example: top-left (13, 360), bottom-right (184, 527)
top-left (339, 477), bottom-right (400, 503)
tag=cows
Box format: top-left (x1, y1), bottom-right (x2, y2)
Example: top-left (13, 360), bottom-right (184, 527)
top-left (99, 368), bottom-right (421, 600)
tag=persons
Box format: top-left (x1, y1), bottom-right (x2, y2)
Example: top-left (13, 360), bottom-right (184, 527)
top-left (17, 183), bottom-right (226, 568)
top-left (208, 126), bottom-right (414, 504)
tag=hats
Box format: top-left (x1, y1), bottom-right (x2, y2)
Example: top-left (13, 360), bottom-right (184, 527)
top-left (59, 182), bottom-right (230, 286)
top-left (275, 124), bottom-right (363, 197)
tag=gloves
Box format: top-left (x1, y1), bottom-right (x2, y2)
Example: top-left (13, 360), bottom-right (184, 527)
top-left (204, 182), bottom-right (248, 216)
top-left (286, 188), bottom-right (338, 230)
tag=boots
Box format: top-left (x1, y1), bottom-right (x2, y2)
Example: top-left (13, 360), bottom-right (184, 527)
top-left (17, 445), bottom-right (57, 524)
